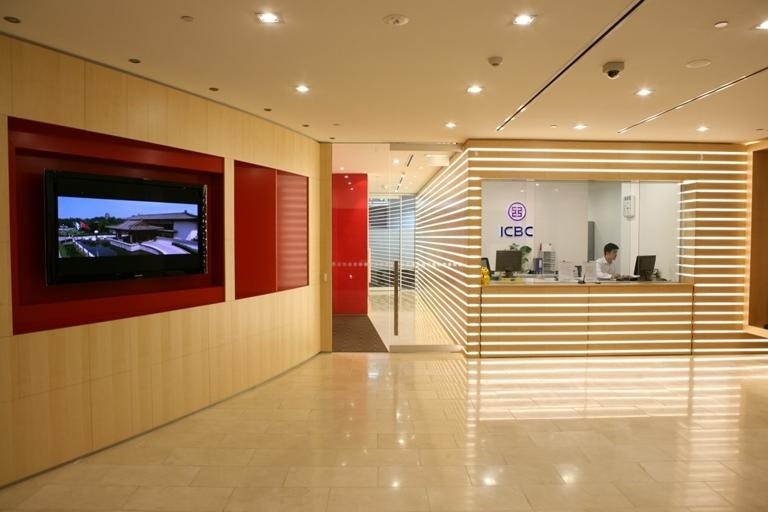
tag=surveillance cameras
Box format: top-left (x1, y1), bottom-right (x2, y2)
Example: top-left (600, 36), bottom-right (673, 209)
top-left (603, 60), bottom-right (624, 81)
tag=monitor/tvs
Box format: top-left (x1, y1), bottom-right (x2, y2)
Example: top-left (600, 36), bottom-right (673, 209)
top-left (43, 169), bottom-right (209, 289)
top-left (634, 254), bottom-right (656, 282)
top-left (495, 251), bottom-right (522, 278)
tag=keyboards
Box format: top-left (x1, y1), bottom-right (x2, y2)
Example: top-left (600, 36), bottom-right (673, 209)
top-left (616, 276), bottom-right (639, 282)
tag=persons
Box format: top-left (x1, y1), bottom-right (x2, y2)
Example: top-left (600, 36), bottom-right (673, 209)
top-left (596, 243), bottom-right (621, 281)
